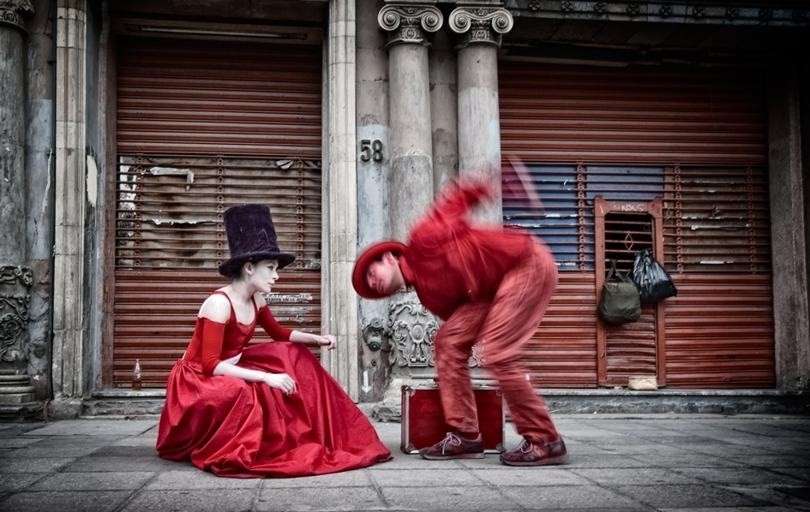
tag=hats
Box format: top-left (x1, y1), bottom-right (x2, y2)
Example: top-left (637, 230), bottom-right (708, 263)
top-left (216, 202), bottom-right (296, 279)
top-left (351, 240), bottom-right (408, 299)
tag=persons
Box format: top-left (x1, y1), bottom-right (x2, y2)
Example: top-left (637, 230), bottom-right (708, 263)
top-left (156, 258), bottom-right (392, 478)
top-left (351, 181), bottom-right (568, 466)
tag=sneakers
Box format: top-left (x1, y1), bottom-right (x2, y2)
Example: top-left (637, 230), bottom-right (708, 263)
top-left (418, 432), bottom-right (487, 460)
top-left (500, 437), bottom-right (567, 465)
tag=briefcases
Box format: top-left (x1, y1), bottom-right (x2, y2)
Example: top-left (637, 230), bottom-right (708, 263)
top-left (399, 375), bottom-right (507, 455)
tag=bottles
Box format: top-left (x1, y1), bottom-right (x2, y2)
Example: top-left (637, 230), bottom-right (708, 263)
top-left (132, 359), bottom-right (142, 391)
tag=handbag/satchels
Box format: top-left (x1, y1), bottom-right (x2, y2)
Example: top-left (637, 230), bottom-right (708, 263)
top-left (597, 259), bottom-right (642, 324)
